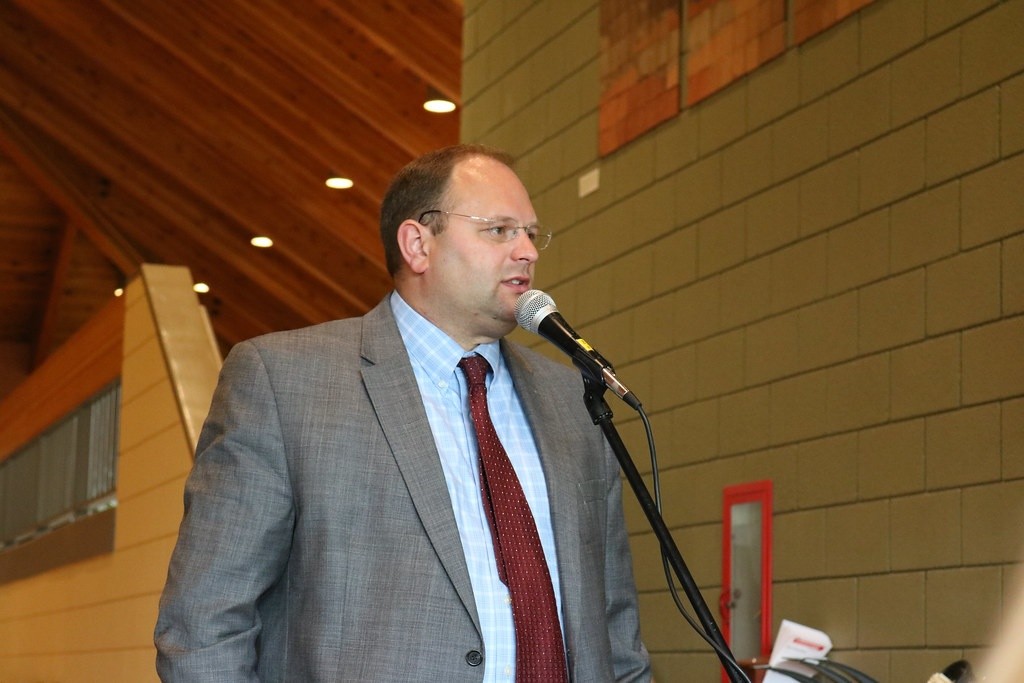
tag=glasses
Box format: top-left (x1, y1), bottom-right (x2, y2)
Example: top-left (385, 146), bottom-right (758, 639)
top-left (419, 210), bottom-right (552, 251)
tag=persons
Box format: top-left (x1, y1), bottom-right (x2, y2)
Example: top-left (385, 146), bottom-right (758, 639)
top-left (155, 141), bottom-right (653, 682)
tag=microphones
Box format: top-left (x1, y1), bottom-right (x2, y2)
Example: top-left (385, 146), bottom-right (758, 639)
top-left (514, 290), bottom-right (642, 411)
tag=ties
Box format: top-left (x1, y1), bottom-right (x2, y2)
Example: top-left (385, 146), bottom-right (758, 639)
top-left (460, 354), bottom-right (567, 683)
